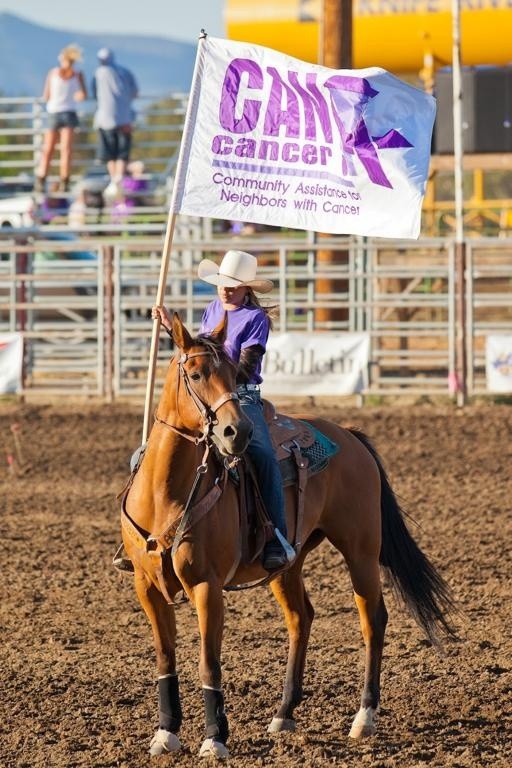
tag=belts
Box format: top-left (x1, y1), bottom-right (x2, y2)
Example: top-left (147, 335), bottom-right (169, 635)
top-left (235, 383), bottom-right (260, 391)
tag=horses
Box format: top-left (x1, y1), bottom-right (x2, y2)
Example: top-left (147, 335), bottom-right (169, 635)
top-left (120, 310), bottom-right (467, 762)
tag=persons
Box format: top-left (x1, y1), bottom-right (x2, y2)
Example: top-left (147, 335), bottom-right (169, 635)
top-left (110, 247), bottom-right (288, 571)
top-left (85, 47), bottom-right (139, 202)
top-left (30, 43), bottom-right (86, 190)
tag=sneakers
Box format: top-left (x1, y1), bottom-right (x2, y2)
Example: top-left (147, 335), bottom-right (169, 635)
top-left (114, 558), bottom-right (133, 571)
top-left (104, 181), bottom-right (120, 195)
top-left (263, 539), bottom-right (286, 569)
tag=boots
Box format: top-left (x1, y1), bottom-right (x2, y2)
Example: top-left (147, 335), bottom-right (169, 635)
top-left (33, 173), bottom-right (68, 192)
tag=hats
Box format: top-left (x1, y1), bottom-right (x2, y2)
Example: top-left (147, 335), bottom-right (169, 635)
top-left (197, 250), bottom-right (274, 294)
top-left (60, 47), bottom-right (80, 61)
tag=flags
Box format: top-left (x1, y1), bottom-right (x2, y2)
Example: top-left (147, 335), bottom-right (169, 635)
top-left (173, 34), bottom-right (440, 241)
